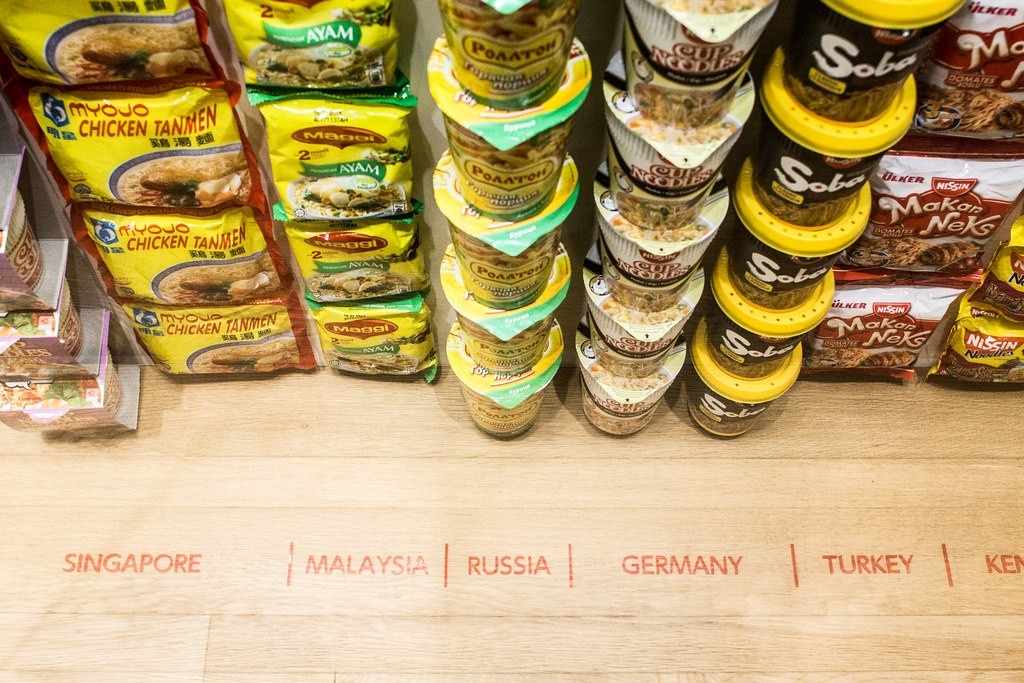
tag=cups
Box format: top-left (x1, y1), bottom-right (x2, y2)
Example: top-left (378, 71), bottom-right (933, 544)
top-left (682, 315), bottom-right (804, 440)
top-left (779, 0), bottom-right (965, 124)
top-left (726, 156), bottom-right (873, 310)
top-left (751, 44), bottom-right (918, 227)
top-left (706, 245), bottom-right (835, 379)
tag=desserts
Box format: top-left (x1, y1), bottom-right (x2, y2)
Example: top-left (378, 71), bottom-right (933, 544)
top-left (809, 349), bottom-right (870, 367)
top-left (924, 242), bottom-right (977, 265)
top-left (865, 351), bottom-right (912, 366)
top-left (859, 238), bottom-right (928, 267)
